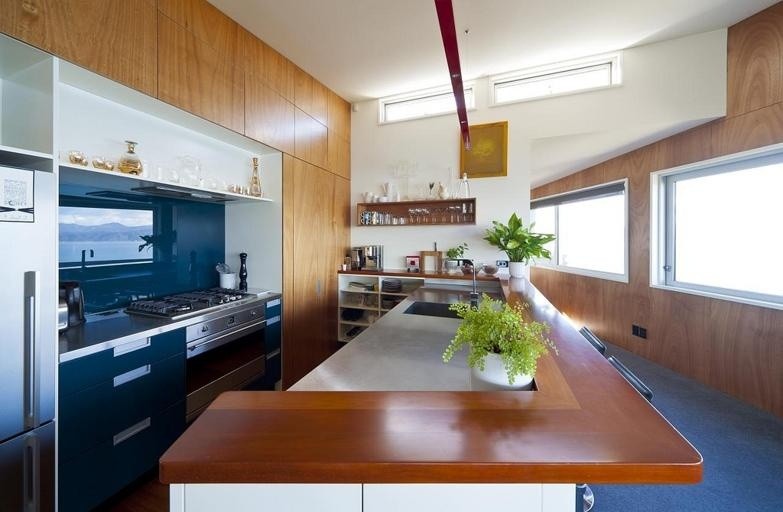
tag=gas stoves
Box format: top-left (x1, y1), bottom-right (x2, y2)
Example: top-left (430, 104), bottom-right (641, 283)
top-left (123, 286), bottom-right (267, 343)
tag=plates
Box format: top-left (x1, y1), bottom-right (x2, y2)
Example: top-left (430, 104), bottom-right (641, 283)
top-left (381, 278), bottom-right (403, 293)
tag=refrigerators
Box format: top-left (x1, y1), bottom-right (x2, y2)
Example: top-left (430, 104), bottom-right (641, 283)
top-left (0, 164), bottom-right (63, 511)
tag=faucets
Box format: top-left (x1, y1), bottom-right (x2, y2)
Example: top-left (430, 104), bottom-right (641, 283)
top-left (458, 258), bottom-right (479, 299)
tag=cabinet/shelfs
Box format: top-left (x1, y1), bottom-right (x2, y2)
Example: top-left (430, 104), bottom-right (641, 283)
top-left (335, 271), bottom-right (424, 343)
top-left (355, 198), bottom-right (476, 227)
top-left (2, 0), bottom-right (350, 179)
top-left (292, 158), bottom-right (336, 383)
top-left (56, 325), bottom-right (187, 512)
top-left (263, 296), bottom-right (281, 386)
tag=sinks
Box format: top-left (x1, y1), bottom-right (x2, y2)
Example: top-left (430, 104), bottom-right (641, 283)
top-left (404, 301), bottom-right (459, 318)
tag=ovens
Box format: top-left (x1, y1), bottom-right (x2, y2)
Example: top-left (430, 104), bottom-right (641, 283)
top-left (185, 316), bottom-right (265, 395)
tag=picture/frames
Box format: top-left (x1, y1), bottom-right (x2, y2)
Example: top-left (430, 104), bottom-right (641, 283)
top-left (460, 119), bottom-right (509, 179)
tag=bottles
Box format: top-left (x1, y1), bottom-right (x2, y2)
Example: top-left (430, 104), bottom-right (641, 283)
top-left (359, 203), bottom-right (474, 226)
top-left (70, 139), bottom-right (264, 197)
top-left (458, 170), bottom-right (472, 200)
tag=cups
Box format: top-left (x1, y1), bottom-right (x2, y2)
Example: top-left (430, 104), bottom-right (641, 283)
top-left (370, 194), bottom-right (391, 203)
top-left (218, 270), bottom-right (238, 290)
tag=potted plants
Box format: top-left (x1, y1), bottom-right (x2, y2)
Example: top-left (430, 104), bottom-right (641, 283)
top-left (447, 294), bottom-right (555, 391)
top-left (445, 249), bottom-right (460, 272)
top-left (487, 211), bottom-right (556, 279)
